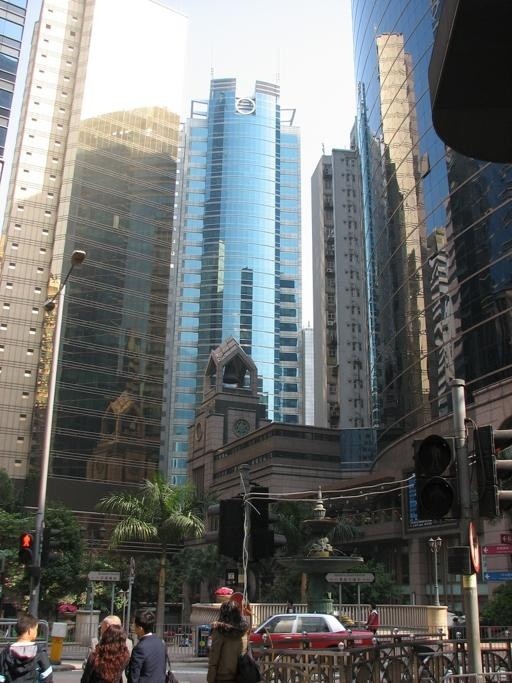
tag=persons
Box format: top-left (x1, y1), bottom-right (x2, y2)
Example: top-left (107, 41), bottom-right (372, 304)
top-left (364, 602), bottom-right (380, 635)
top-left (79, 609), bottom-right (168, 683)
top-left (0, 614), bottom-right (54, 683)
top-left (453, 617), bottom-right (461, 639)
top-left (208, 601), bottom-right (248, 683)
top-left (285, 598), bottom-right (295, 614)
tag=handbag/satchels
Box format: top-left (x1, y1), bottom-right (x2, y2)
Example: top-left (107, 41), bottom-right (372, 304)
top-left (235, 646), bottom-right (262, 682)
top-left (164, 649), bottom-right (178, 682)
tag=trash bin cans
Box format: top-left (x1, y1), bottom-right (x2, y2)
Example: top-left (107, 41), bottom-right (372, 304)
top-left (197, 625), bottom-right (212, 657)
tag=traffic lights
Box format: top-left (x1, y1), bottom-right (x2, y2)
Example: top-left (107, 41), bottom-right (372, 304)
top-left (205, 498), bottom-right (244, 563)
top-left (249, 485), bottom-right (287, 561)
top-left (413, 433), bottom-right (459, 522)
top-left (41, 528), bottom-right (62, 568)
top-left (18, 531), bottom-right (35, 564)
top-left (473, 424), bottom-right (512, 518)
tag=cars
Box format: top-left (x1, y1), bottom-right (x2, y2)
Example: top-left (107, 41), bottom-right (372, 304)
top-left (246, 613), bottom-right (380, 672)
top-left (447, 609), bottom-right (510, 643)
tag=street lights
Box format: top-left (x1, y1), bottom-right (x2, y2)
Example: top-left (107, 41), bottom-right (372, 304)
top-left (28, 245), bottom-right (86, 619)
top-left (428, 536), bottom-right (442, 606)
top-left (119, 589), bottom-right (130, 629)
